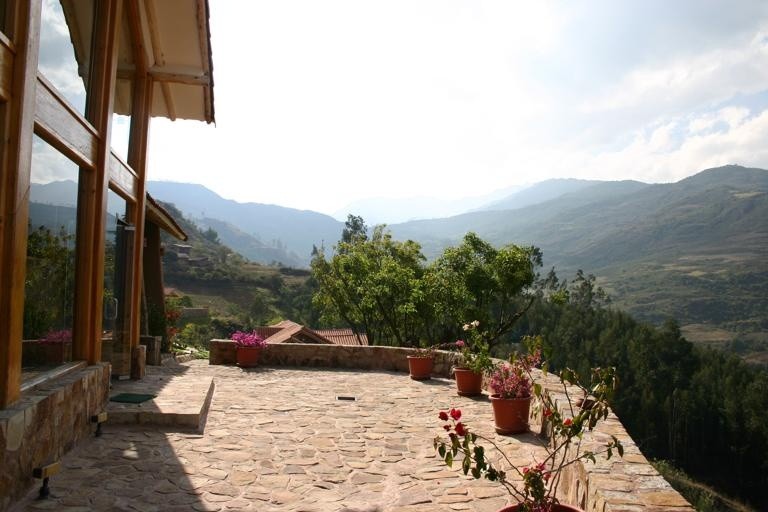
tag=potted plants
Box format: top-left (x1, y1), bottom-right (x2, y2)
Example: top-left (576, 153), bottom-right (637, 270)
top-left (409, 341), bottom-right (448, 358)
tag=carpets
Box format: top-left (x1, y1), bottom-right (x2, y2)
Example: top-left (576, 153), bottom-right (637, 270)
top-left (110, 393), bottom-right (155, 403)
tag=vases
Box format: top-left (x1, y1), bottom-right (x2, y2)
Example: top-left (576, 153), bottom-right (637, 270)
top-left (498, 503), bottom-right (584, 512)
top-left (406, 355), bottom-right (434, 381)
top-left (488, 393), bottom-right (534, 434)
top-left (453, 367), bottom-right (482, 396)
top-left (236, 346), bottom-right (261, 366)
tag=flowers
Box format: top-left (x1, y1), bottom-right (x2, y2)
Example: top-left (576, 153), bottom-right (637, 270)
top-left (434, 360), bottom-right (623, 512)
top-left (37, 326), bottom-right (73, 345)
top-left (480, 335), bottom-right (544, 398)
top-left (452, 321), bottom-right (492, 375)
top-left (230, 329), bottom-right (268, 350)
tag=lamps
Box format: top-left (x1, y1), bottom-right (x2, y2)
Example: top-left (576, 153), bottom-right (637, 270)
top-left (117, 218), bottom-right (136, 231)
